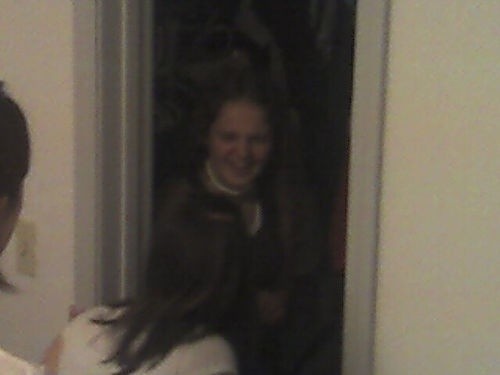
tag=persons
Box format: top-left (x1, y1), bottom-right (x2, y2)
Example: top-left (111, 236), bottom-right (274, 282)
top-left (40, 195), bottom-right (246, 374)
top-left (150, 84), bottom-right (324, 375)
top-left (0, 81), bottom-right (47, 375)
